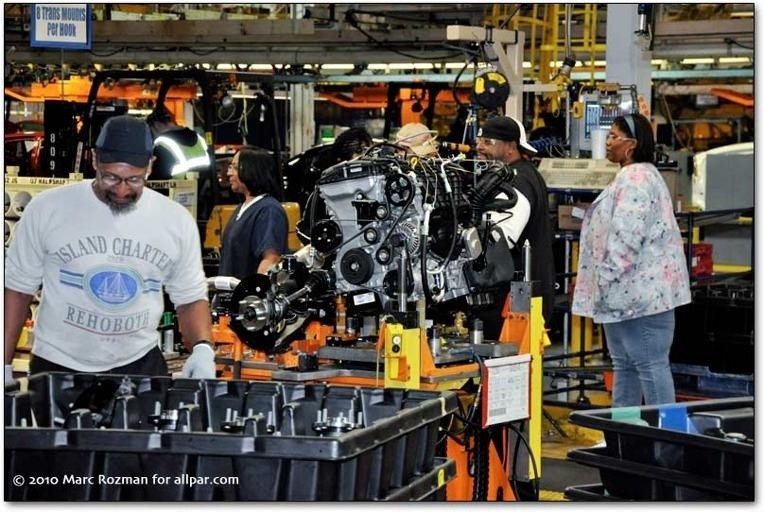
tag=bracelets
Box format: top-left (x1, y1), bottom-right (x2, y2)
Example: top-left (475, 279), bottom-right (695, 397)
top-left (191, 340), bottom-right (214, 349)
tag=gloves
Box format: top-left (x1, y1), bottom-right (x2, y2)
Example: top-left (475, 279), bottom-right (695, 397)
top-left (181, 343), bottom-right (218, 379)
top-left (4, 364), bottom-right (17, 388)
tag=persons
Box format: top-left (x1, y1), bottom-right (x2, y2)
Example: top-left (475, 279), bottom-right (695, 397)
top-left (462, 116), bottom-right (555, 340)
top-left (144, 106), bottom-right (213, 218)
top-left (5, 115), bottom-right (217, 386)
top-left (210, 145), bottom-right (288, 307)
top-left (294, 126), bottom-right (374, 246)
top-left (571, 113), bottom-right (692, 408)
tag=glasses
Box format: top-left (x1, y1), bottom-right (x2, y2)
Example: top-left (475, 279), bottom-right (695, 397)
top-left (228, 163), bottom-right (240, 172)
top-left (475, 135), bottom-right (496, 146)
top-left (605, 133), bottom-right (633, 143)
top-left (95, 165), bottom-right (149, 188)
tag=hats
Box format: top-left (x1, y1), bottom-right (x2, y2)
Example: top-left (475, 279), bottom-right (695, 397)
top-left (146, 109), bottom-right (170, 122)
top-left (94, 114), bottom-right (153, 168)
top-left (477, 115), bottom-right (520, 142)
top-left (506, 115), bottom-right (538, 154)
